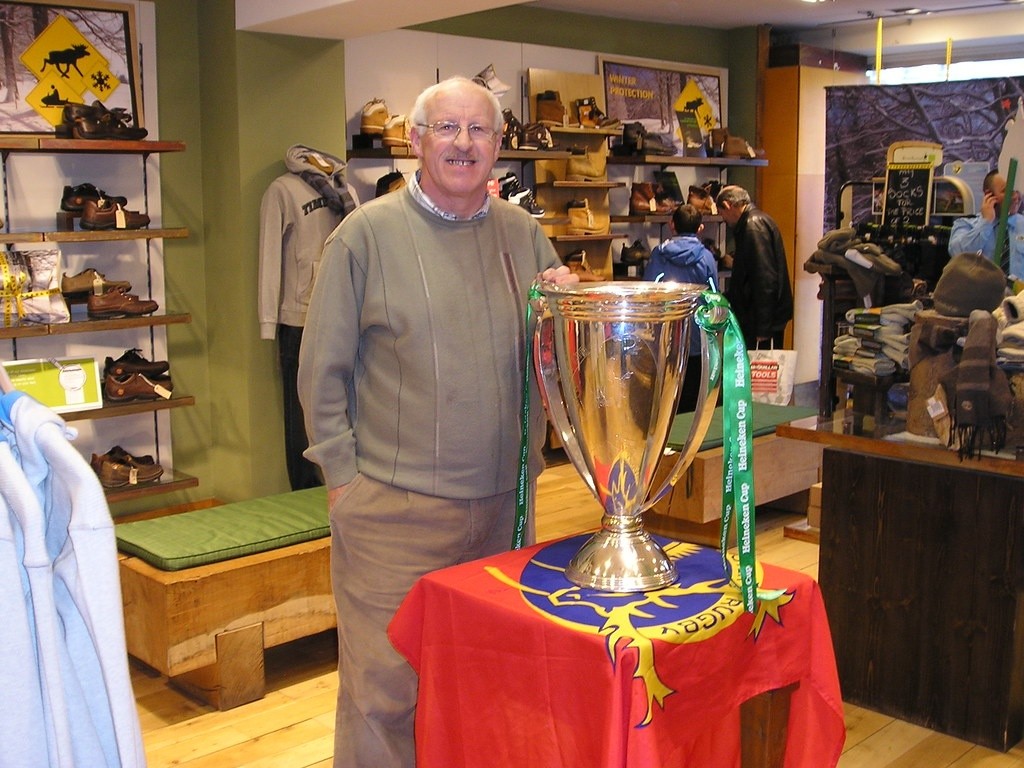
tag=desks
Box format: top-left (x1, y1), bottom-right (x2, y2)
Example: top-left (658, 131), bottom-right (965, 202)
top-left (776, 416), bottom-right (1024, 754)
top-left (386, 533), bottom-right (848, 768)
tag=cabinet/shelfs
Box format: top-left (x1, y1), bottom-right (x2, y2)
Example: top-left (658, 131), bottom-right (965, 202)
top-left (607, 155), bottom-right (769, 277)
top-left (527, 68), bottom-right (628, 448)
top-left (0, 138), bottom-right (200, 503)
top-left (346, 147), bottom-right (573, 224)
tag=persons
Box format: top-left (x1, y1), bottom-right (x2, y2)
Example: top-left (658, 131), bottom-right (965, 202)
top-left (258, 144), bottom-right (361, 492)
top-left (716, 185), bottom-right (794, 349)
top-left (642, 204), bottom-right (719, 413)
top-left (297, 77), bottom-right (580, 768)
top-left (948, 169), bottom-right (1024, 281)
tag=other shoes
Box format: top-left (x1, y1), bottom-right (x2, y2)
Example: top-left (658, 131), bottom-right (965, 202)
top-left (375, 168), bottom-right (407, 197)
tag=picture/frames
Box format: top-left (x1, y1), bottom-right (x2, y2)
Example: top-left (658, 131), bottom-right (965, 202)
top-left (0, 0), bottom-right (144, 138)
top-left (598, 53), bottom-right (728, 158)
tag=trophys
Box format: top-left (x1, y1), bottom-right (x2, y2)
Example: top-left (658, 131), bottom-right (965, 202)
top-left (528, 273), bottom-right (721, 592)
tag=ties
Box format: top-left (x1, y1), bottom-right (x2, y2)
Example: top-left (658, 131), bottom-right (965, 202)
top-left (998, 222), bottom-right (1010, 276)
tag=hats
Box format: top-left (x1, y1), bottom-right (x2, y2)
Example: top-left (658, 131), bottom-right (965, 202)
top-left (933, 249), bottom-right (1007, 317)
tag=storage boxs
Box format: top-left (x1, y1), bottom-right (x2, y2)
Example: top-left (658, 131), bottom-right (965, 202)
top-left (767, 43), bottom-right (868, 72)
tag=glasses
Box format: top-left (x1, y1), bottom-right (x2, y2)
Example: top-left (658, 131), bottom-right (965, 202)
top-left (418, 120), bottom-right (497, 140)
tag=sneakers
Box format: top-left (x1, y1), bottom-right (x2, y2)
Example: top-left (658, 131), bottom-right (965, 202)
top-left (705, 129), bottom-right (765, 159)
top-left (498, 172), bottom-right (545, 218)
top-left (360, 97), bottom-right (411, 159)
top-left (622, 121), bottom-right (678, 155)
top-left (502, 108), bottom-right (560, 151)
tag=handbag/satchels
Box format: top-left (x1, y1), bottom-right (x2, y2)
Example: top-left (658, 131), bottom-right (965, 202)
top-left (747, 337), bottom-right (798, 406)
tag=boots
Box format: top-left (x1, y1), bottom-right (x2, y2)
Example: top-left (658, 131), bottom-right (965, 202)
top-left (687, 181), bottom-right (723, 215)
top-left (566, 198), bottom-right (606, 235)
top-left (565, 144), bottom-right (605, 181)
top-left (629, 182), bottom-right (684, 216)
top-left (563, 249), bottom-right (607, 282)
top-left (575, 97), bottom-right (620, 128)
top-left (537, 91), bottom-right (580, 127)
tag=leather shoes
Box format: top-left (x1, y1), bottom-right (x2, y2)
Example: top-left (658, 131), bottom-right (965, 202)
top-left (92, 348), bottom-right (173, 488)
top-left (62, 100), bottom-right (160, 318)
top-left (621, 239), bottom-right (651, 263)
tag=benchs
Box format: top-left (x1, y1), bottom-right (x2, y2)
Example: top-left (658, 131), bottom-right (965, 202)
top-left (114, 459), bottom-right (604, 712)
top-left (641, 380), bottom-right (848, 549)
